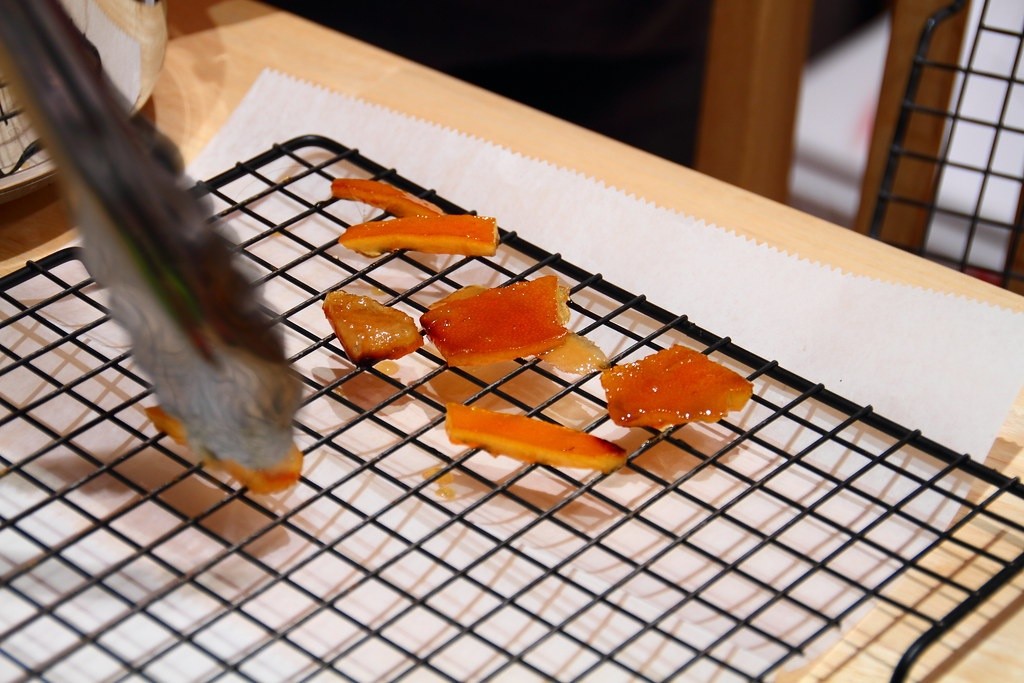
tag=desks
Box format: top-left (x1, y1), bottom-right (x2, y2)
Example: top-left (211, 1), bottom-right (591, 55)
top-left (0, 0), bottom-right (1024, 683)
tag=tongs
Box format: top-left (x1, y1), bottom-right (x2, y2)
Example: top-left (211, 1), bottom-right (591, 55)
top-left (0, 0), bottom-right (305, 474)
